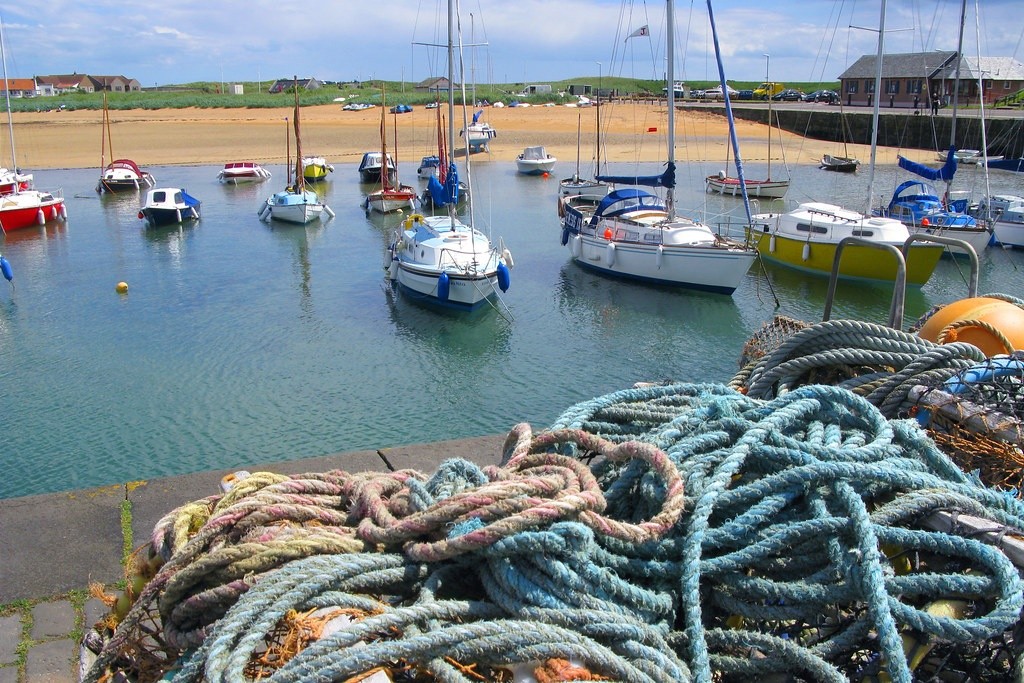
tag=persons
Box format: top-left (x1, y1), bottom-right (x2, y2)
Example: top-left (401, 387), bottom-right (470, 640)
top-left (932, 92), bottom-right (938, 115)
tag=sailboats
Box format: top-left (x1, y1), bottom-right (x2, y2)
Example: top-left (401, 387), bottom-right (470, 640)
top-left (0, 25), bottom-right (69, 232)
top-left (872, 2), bottom-right (996, 265)
top-left (745, 0), bottom-right (946, 291)
top-left (459, 10), bottom-right (498, 154)
top-left (970, 1), bottom-right (1024, 251)
top-left (386, 1), bottom-right (516, 314)
top-left (560, 0), bottom-right (760, 297)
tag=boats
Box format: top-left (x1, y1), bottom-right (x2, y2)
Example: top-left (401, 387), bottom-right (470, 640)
top-left (98, 84), bottom-right (156, 190)
top-left (662, 79), bottom-right (693, 99)
top-left (359, 85), bottom-right (558, 212)
top-left (820, 97), bottom-right (861, 174)
top-left (284, 117), bottom-right (335, 184)
top-left (560, 88), bottom-right (612, 196)
top-left (216, 162), bottom-right (271, 186)
top-left (138, 188), bottom-right (202, 224)
top-left (707, 83), bottom-right (791, 199)
top-left (258, 76), bottom-right (336, 224)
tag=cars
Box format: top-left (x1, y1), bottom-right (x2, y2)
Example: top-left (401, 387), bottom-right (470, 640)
top-left (690, 81), bottom-right (841, 104)
